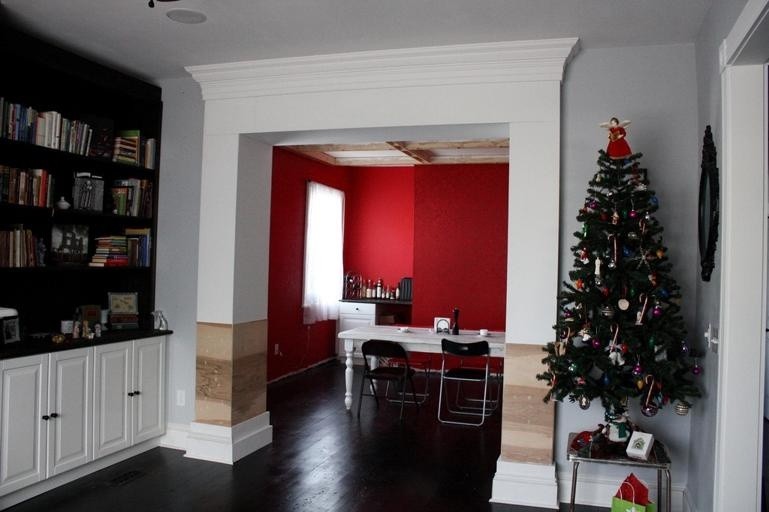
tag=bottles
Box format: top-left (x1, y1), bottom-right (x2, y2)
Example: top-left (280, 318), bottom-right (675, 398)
top-left (361, 277), bottom-right (400, 300)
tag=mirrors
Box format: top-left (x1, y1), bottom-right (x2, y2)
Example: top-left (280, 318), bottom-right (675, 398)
top-left (697, 124), bottom-right (719, 282)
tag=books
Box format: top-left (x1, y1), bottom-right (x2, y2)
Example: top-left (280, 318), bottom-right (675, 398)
top-left (0, 223), bottom-right (37, 268)
top-left (111, 177), bottom-right (152, 219)
top-left (0, 163), bottom-right (54, 209)
top-left (88, 228), bottom-right (151, 267)
top-left (0, 96), bottom-right (156, 170)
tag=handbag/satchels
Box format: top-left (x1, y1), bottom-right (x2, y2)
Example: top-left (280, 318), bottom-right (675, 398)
top-left (611, 479), bottom-right (658, 512)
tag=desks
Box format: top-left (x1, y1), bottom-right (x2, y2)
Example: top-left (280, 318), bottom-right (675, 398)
top-left (338, 297), bottom-right (412, 366)
top-left (338, 324), bottom-right (507, 412)
top-left (567, 432), bottom-right (672, 512)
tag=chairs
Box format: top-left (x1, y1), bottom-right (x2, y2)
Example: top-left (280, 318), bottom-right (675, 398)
top-left (455, 358), bottom-right (502, 411)
top-left (437, 338), bottom-right (493, 427)
top-left (357, 339), bottom-right (417, 423)
top-left (385, 351), bottom-right (432, 405)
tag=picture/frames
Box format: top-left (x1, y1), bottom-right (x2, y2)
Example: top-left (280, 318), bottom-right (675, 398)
top-left (107, 292), bottom-right (139, 315)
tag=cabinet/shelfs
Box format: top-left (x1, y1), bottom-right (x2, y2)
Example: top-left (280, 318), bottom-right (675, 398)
top-left (93, 334), bottom-right (166, 461)
top-left (0, 346), bottom-right (94, 497)
top-left (0, 45), bottom-right (164, 330)
